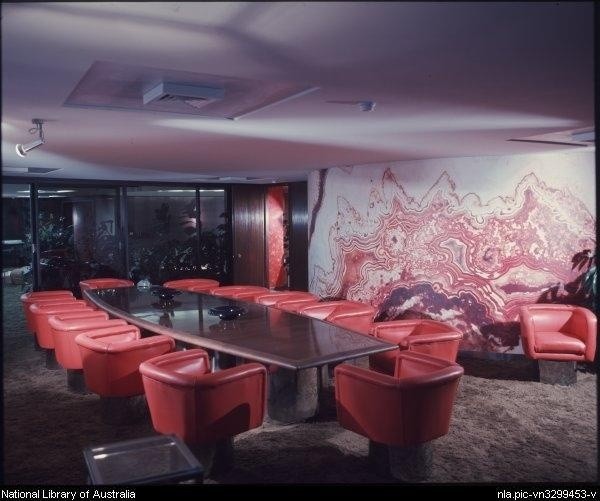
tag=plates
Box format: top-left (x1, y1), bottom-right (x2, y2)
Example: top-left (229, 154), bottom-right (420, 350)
top-left (209, 306), bottom-right (248, 320)
top-left (152, 288), bottom-right (183, 299)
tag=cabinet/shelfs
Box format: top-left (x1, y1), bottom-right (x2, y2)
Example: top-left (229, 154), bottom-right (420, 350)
top-left (230, 183), bottom-right (293, 292)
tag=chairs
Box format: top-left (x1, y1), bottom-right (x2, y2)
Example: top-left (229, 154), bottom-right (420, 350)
top-left (517, 303), bottom-right (597, 386)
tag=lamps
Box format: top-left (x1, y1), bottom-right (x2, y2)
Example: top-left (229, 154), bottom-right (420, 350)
top-left (14, 117), bottom-right (49, 158)
